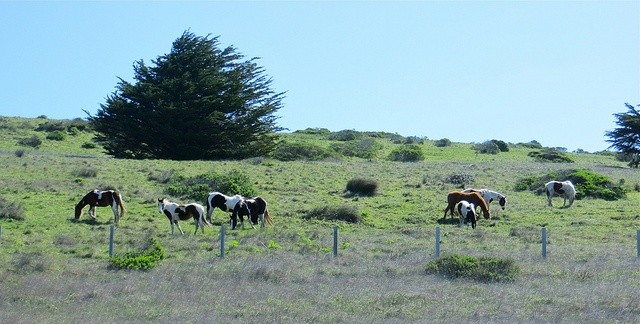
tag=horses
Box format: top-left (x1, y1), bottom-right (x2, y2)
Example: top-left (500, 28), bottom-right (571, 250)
top-left (461, 188), bottom-right (506, 215)
top-left (443, 191), bottom-right (490, 220)
top-left (206, 192), bottom-right (250, 224)
top-left (457, 200), bottom-right (477, 230)
top-left (75, 189), bottom-right (127, 223)
top-left (229, 196), bottom-right (273, 230)
top-left (545, 180), bottom-right (576, 207)
top-left (158, 198), bottom-right (212, 236)
